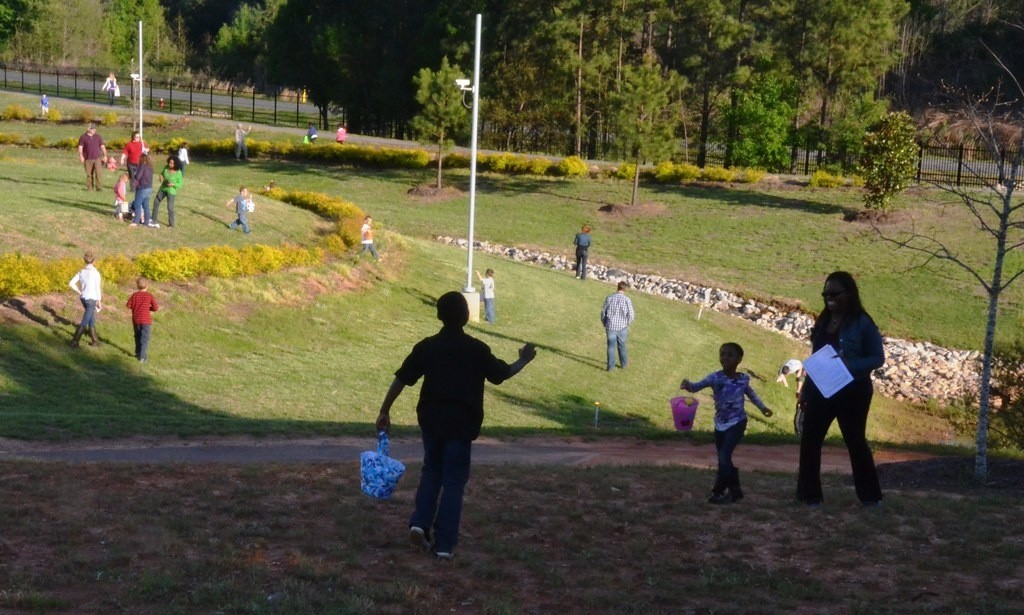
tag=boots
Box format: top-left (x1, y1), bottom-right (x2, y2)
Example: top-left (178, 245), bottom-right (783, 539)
top-left (89, 327), bottom-right (100, 346)
top-left (71, 330), bottom-right (82, 348)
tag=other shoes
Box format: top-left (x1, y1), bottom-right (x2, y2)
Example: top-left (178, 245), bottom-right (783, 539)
top-left (436, 547), bottom-right (454, 558)
top-left (728, 487), bottom-right (743, 502)
top-left (709, 484), bottom-right (731, 504)
top-left (410, 525), bottom-right (430, 547)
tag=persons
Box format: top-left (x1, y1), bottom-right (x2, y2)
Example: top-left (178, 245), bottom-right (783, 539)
top-left (40, 93), bottom-right (49, 117)
top-left (226, 185), bottom-right (251, 233)
top-left (148, 155), bottom-right (182, 228)
top-left (355, 215), bottom-right (379, 259)
top-left (77, 122), bottom-right (107, 192)
top-left (129, 153), bottom-right (152, 227)
top-left (126, 277), bottom-right (158, 362)
top-left (307, 121), bottom-right (318, 144)
top-left (178, 142), bottom-right (188, 178)
top-left (680, 342), bottom-right (773, 506)
top-left (120, 131), bottom-right (150, 192)
top-left (601, 281), bottom-right (634, 373)
top-left (778, 359), bottom-right (803, 397)
top-left (113, 173), bottom-right (129, 221)
top-left (475, 268), bottom-right (496, 323)
top-left (794, 270), bottom-right (884, 513)
top-left (376, 292), bottom-right (537, 560)
top-left (336, 122), bottom-right (346, 144)
top-left (573, 223), bottom-right (590, 279)
top-left (101, 71), bottom-right (118, 106)
top-left (233, 123), bottom-right (252, 163)
top-left (69, 253), bottom-right (102, 347)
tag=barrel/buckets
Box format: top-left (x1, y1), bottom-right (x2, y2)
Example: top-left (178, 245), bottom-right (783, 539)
top-left (671, 384), bottom-right (699, 431)
top-left (359, 430), bottom-right (406, 499)
top-left (365, 228), bottom-right (375, 240)
top-left (119, 198), bottom-right (129, 212)
top-left (247, 195), bottom-right (255, 213)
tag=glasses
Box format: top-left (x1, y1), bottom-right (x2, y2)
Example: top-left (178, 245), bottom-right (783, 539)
top-left (822, 288), bottom-right (847, 298)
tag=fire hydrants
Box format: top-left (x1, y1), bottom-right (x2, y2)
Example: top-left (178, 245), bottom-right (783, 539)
top-left (159, 97), bottom-right (164, 108)
